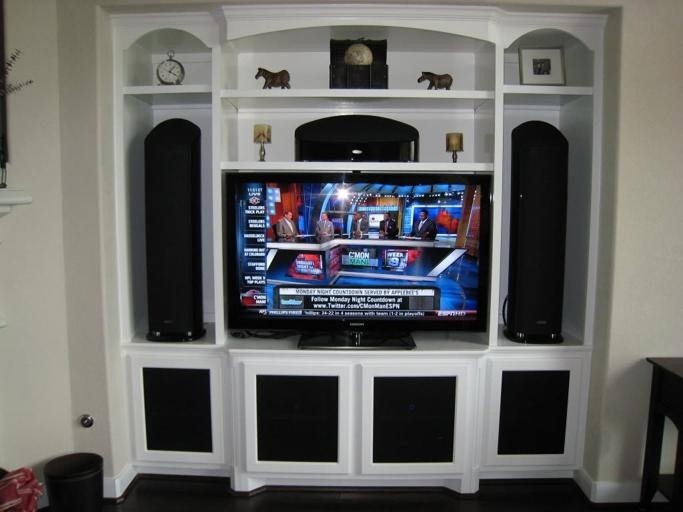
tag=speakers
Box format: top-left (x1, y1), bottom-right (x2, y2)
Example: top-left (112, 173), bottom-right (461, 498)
top-left (144, 117), bottom-right (202, 339)
top-left (294, 116), bottom-right (421, 163)
top-left (506, 119), bottom-right (569, 342)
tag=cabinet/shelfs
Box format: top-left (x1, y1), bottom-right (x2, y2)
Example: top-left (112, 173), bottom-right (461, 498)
top-left (107, 0), bottom-right (610, 498)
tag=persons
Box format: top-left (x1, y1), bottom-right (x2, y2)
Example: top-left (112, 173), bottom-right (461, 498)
top-left (275, 211), bottom-right (300, 243)
top-left (350, 210), bottom-right (369, 239)
top-left (378, 212), bottom-right (400, 240)
top-left (410, 209), bottom-right (437, 241)
top-left (315, 212), bottom-right (335, 243)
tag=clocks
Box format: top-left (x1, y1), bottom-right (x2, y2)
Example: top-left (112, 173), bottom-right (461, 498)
top-left (155, 45), bottom-right (188, 85)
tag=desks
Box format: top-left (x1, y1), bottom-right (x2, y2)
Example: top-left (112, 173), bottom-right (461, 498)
top-left (638, 353), bottom-right (683, 512)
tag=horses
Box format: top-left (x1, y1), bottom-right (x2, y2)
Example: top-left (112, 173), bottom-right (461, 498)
top-left (416, 71), bottom-right (453, 91)
top-left (254, 67), bottom-right (291, 90)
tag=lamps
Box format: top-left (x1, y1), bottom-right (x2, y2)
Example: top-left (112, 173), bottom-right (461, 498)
top-left (443, 130), bottom-right (467, 165)
top-left (248, 121), bottom-right (275, 163)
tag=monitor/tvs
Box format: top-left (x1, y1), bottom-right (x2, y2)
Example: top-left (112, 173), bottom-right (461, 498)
top-left (223, 168), bottom-right (488, 348)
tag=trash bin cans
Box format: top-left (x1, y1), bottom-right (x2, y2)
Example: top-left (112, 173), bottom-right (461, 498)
top-left (43, 453), bottom-right (104, 512)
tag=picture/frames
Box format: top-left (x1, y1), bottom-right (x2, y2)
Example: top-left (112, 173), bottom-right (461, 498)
top-left (517, 43), bottom-right (567, 89)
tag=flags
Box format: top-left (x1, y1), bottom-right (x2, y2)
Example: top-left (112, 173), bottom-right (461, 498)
top-left (433, 211), bottom-right (459, 232)
